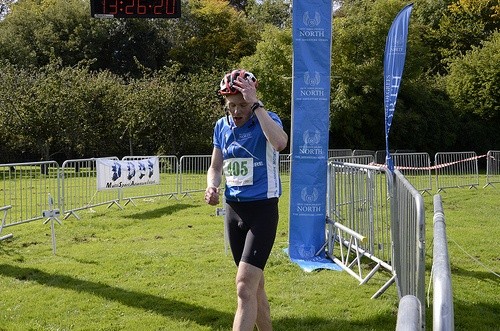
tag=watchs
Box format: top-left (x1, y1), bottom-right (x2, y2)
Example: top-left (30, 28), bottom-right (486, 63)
top-left (251, 99), bottom-right (264, 112)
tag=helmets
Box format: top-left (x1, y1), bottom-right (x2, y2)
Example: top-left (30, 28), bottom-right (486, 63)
top-left (217, 70), bottom-right (258, 95)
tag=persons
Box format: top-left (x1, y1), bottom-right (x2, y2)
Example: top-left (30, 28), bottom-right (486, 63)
top-left (205, 68), bottom-right (289, 331)
